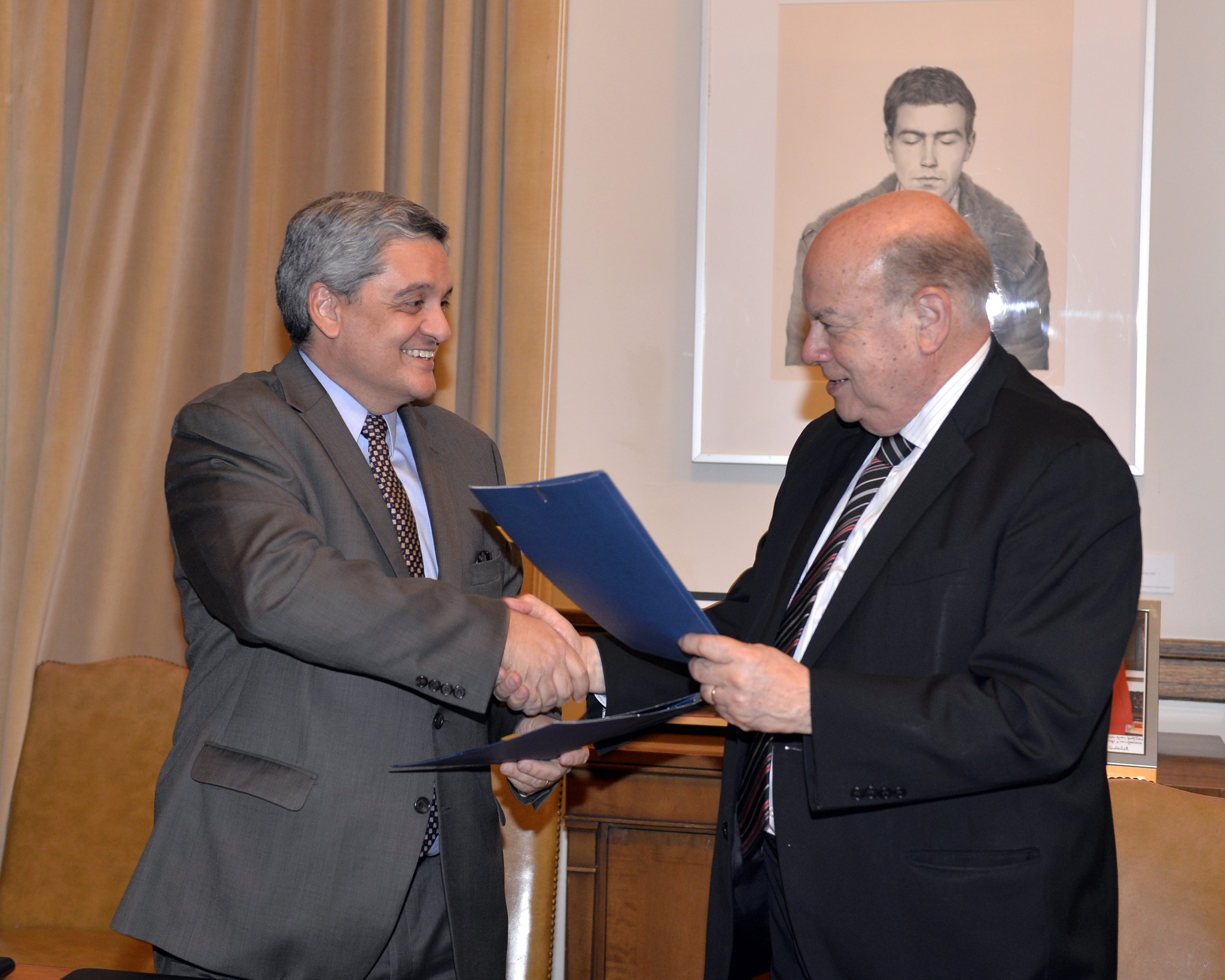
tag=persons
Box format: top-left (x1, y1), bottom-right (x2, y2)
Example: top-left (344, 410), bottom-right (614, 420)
top-left (491, 190), bottom-right (1145, 980)
top-left (109, 186), bottom-right (593, 978)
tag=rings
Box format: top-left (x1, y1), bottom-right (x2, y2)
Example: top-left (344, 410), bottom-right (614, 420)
top-left (710, 687), bottom-right (723, 706)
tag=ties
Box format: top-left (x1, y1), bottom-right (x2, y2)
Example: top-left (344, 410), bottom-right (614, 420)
top-left (360, 415), bottom-right (439, 863)
top-left (736, 433), bottom-right (917, 872)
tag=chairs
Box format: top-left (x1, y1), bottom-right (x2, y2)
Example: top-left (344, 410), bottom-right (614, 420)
top-left (1107, 778), bottom-right (1225, 980)
top-left (0, 657), bottom-right (188, 980)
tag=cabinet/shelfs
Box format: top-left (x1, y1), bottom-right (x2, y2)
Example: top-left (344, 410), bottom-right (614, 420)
top-left (566, 732), bottom-right (1224, 980)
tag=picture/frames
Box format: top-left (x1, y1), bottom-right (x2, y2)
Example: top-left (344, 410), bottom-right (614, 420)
top-left (1103, 599), bottom-right (1161, 785)
top-left (691, 0), bottom-right (1159, 477)
